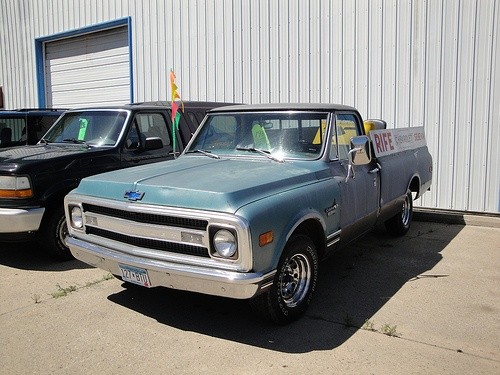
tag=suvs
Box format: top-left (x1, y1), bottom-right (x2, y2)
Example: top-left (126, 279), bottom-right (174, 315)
top-left (0, 107), bottom-right (72, 145)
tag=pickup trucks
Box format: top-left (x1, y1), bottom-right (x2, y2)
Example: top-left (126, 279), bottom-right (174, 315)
top-left (1, 100), bottom-right (246, 256)
top-left (63, 103), bottom-right (433, 325)
